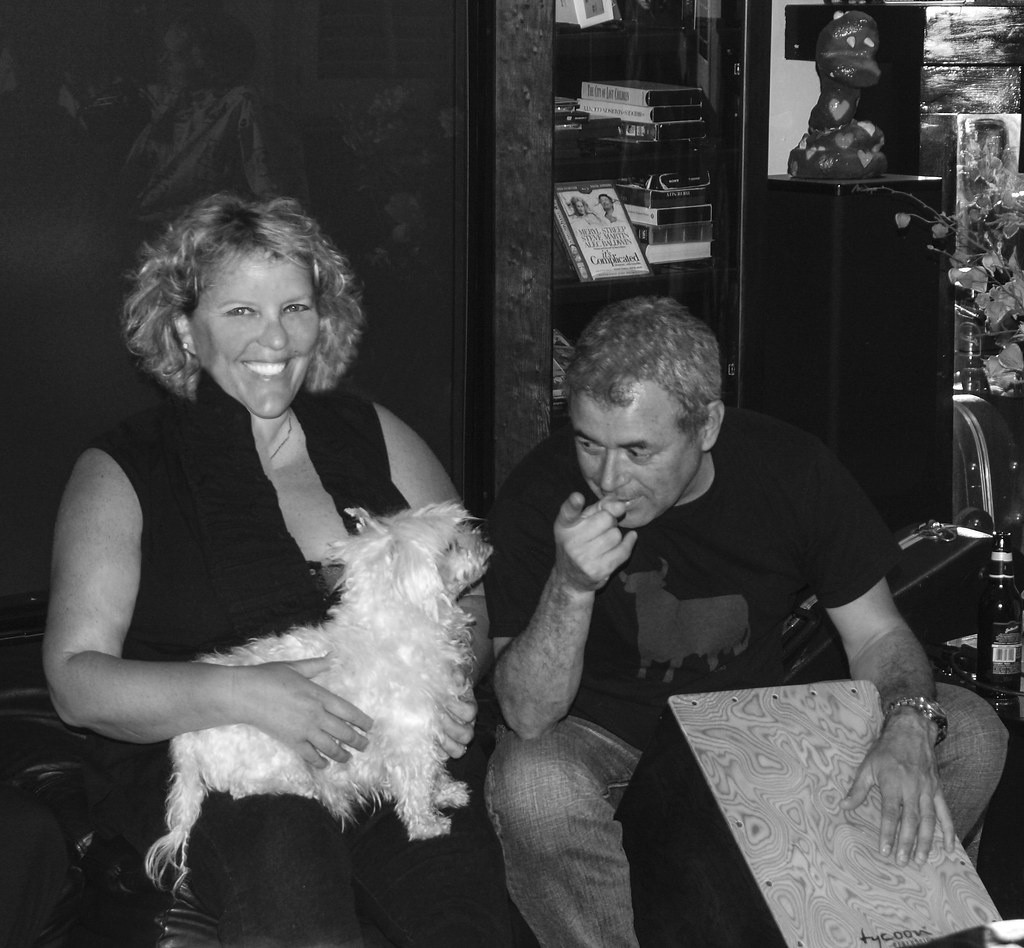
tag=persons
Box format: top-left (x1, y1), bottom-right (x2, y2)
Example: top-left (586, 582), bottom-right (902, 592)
top-left (41, 186), bottom-right (515, 948)
top-left (486, 293), bottom-right (1011, 948)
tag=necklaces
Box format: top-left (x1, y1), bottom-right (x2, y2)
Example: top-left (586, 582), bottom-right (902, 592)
top-left (261, 410), bottom-right (293, 465)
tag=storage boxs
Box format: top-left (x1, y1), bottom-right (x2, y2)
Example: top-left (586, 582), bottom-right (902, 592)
top-left (550, 78), bottom-right (713, 282)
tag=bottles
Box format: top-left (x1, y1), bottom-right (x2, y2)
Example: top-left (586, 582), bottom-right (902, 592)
top-left (977, 534), bottom-right (1022, 684)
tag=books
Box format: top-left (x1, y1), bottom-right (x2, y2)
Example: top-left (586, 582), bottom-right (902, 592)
top-left (547, 79), bottom-right (720, 285)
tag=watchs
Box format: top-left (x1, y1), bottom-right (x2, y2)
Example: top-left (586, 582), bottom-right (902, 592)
top-left (881, 695), bottom-right (949, 748)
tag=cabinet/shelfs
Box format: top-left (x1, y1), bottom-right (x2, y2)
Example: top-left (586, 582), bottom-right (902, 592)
top-left (494, 3), bottom-right (772, 507)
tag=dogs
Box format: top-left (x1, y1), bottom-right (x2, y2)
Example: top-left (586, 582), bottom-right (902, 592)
top-left (141, 494), bottom-right (497, 903)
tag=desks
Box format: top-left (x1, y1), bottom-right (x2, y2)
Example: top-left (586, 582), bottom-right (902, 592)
top-left (764, 172), bottom-right (955, 534)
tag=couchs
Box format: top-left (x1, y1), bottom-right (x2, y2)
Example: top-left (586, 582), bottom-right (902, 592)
top-left (0, 633), bottom-right (786, 947)
